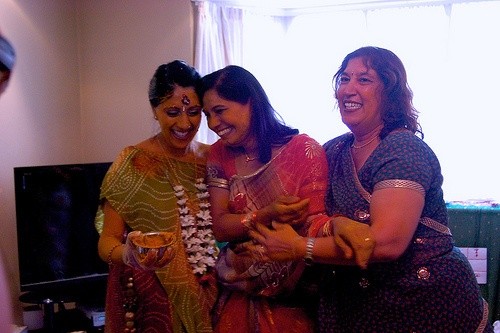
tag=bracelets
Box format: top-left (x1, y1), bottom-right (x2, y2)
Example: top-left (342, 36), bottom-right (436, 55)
top-left (240, 212), bottom-right (257, 233)
top-left (107, 243), bottom-right (125, 266)
top-left (323, 219), bottom-right (332, 236)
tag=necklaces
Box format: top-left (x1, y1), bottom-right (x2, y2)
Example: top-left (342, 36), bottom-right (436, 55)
top-left (351, 135), bottom-right (379, 148)
top-left (242, 152), bottom-right (258, 166)
top-left (156, 134), bottom-right (216, 282)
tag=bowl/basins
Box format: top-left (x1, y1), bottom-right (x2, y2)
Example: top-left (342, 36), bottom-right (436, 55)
top-left (131, 232), bottom-right (178, 268)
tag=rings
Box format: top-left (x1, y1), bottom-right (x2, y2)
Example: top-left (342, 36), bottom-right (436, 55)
top-left (259, 245), bottom-right (265, 253)
top-left (365, 237), bottom-right (371, 241)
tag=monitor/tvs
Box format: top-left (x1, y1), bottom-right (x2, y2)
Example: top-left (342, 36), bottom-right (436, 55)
top-left (13, 162), bottom-right (112, 307)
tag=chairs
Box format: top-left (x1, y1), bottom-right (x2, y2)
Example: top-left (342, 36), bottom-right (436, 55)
top-left (444, 204), bottom-right (500, 323)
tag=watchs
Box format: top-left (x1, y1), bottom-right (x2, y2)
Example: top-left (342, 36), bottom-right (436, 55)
top-left (304, 238), bottom-right (314, 266)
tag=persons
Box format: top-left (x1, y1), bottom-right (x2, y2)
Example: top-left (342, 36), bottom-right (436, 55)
top-left (202, 65), bottom-right (373, 333)
top-left (244, 46), bottom-right (492, 333)
top-left (95, 59), bottom-right (219, 333)
